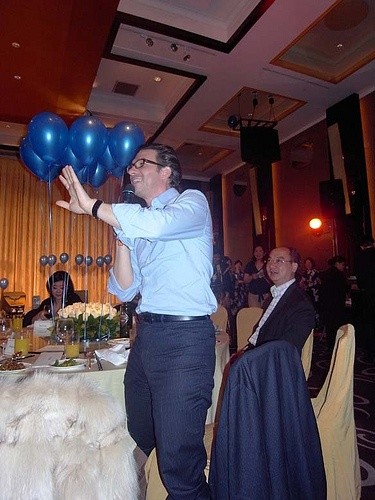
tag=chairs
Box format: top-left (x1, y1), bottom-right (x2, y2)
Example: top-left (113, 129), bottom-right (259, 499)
top-left (144, 302), bottom-right (361, 500)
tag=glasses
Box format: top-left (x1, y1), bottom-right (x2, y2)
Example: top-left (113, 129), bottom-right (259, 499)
top-left (126, 159), bottom-right (162, 174)
top-left (263, 258), bottom-right (292, 265)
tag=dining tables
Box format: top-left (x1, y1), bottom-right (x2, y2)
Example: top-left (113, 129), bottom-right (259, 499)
top-left (0, 338), bottom-right (148, 500)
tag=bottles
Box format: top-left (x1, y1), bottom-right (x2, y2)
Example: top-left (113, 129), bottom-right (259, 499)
top-left (120, 302), bottom-right (132, 338)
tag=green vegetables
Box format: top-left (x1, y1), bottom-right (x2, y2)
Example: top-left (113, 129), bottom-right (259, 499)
top-left (52, 358), bottom-right (77, 368)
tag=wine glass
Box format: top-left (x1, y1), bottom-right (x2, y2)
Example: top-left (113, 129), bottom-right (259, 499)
top-left (57, 318), bottom-right (75, 359)
top-left (0, 318), bottom-right (12, 356)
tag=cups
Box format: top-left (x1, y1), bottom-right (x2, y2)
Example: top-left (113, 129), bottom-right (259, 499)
top-left (13, 314), bottom-right (23, 331)
top-left (13, 328), bottom-right (28, 358)
top-left (44, 304), bottom-right (53, 319)
top-left (66, 330), bottom-right (80, 359)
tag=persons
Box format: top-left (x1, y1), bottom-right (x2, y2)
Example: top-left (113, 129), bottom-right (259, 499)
top-left (210, 237), bottom-right (375, 344)
top-left (55, 144), bottom-right (219, 500)
top-left (22, 271), bottom-right (82, 327)
top-left (233, 244), bottom-right (318, 362)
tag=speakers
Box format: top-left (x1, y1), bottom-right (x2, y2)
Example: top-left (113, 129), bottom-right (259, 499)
top-left (318, 177), bottom-right (345, 220)
top-left (239, 128), bottom-right (280, 171)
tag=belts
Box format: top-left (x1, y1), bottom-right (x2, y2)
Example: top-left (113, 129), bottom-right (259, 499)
top-left (139, 313), bottom-right (210, 323)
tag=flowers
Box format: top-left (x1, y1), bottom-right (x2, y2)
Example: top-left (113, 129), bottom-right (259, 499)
top-left (51, 301), bottom-right (121, 342)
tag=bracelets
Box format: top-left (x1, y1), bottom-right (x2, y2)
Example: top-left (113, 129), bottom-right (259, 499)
top-left (92, 199), bottom-right (103, 219)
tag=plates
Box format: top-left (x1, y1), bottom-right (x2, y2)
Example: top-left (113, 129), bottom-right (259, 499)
top-left (0, 362), bottom-right (32, 374)
top-left (48, 357), bottom-right (87, 369)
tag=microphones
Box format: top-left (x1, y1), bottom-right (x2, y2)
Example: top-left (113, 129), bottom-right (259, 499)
top-left (124, 183), bottom-right (135, 204)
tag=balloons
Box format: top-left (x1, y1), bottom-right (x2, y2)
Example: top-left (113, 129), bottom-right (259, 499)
top-left (85, 256), bottom-right (92, 265)
top-left (39, 256), bottom-right (47, 266)
top-left (104, 255), bottom-right (112, 264)
top-left (96, 256), bottom-right (104, 266)
top-left (75, 254), bottom-right (83, 265)
top-left (48, 255), bottom-right (56, 265)
top-left (60, 253), bottom-right (68, 264)
top-left (18, 111), bottom-right (147, 189)
top-left (0, 278), bottom-right (9, 289)
top-left (228, 116), bottom-right (237, 127)
